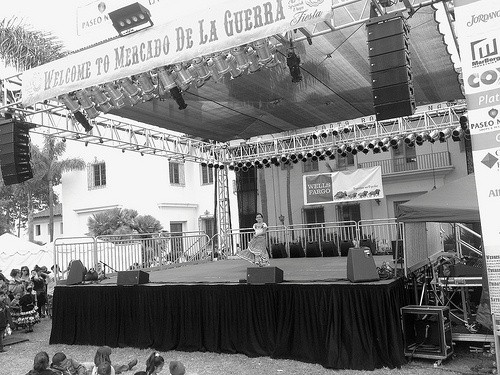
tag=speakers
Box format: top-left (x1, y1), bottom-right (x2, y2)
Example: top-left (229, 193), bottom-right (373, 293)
top-left (0, 122), bottom-right (33, 185)
top-left (118, 271), bottom-right (149, 286)
top-left (272, 240), bottom-right (377, 259)
top-left (247, 267), bottom-right (283, 284)
top-left (347, 248), bottom-right (380, 282)
top-left (68, 260), bottom-right (85, 285)
top-left (367, 17), bottom-right (416, 121)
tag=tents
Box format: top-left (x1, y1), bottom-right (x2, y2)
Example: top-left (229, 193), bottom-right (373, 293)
top-left (0, 233), bottom-right (56, 282)
top-left (395, 171), bottom-right (480, 279)
top-left (39, 231), bottom-right (117, 278)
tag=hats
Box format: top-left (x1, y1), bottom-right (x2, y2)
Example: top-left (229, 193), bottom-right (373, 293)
top-left (169, 360), bottom-right (186, 375)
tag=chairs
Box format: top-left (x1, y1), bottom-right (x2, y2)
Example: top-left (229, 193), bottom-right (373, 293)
top-left (269, 238), bottom-right (376, 259)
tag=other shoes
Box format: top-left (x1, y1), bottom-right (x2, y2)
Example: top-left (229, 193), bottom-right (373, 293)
top-left (128, 359), bottom-right (137, 370)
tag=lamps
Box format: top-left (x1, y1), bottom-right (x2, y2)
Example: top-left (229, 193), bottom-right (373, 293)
top-left (201, 112), bottom-right (470, 173)
top-left (57, 31), bottom-right (304, 132)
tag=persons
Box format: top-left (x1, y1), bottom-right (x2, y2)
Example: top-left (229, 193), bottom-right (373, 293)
top-left (236, 212), bottom-right (271, 267)
top-left (49, 352), bottom-right (138, 375)
top-left (91, 346), bottom-right (116, 375)
top-left (146, 351), bottom-right (165, 375)
top-left (0, 262), bottom-right (105, 329)
top-left (24, 351), bottom-right (60, 375)
